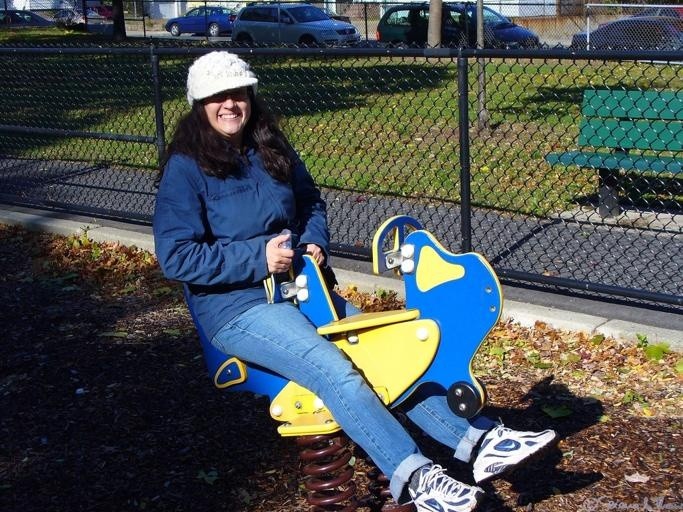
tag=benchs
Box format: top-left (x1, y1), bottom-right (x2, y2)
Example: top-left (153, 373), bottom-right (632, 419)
top-left (543, 88), bottom-right (683, 221)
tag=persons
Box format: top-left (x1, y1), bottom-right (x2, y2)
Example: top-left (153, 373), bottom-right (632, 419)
top-left (152, 51), bottom-right (557, 512)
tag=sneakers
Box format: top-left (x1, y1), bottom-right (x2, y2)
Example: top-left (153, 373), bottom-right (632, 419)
top-left (472, 421), bottom-right (557, 484)
top-left (406, 463), bottom-right (486, 512)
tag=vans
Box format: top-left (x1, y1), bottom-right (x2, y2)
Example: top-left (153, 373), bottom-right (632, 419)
top-left (229, 4), bottom-right (360, 47)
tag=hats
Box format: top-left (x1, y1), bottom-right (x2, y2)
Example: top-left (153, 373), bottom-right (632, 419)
top-left (185, 50), bottom-right (260, 109)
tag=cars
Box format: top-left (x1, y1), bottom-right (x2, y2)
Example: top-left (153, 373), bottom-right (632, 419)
top-left (570, 17), bottom-right (683, 52)
top-left (49, 9), bottom-right (107, 25)
top-left (164, 6), bottom-right (237, 37)
top-left (319, 7), bottom-right (353, 25)
top-left (596, 7), bottom-right (683, 29)
top-left (0, 10), bottom-right (60, 26)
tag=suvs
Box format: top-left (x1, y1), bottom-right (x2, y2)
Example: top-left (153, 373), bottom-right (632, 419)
top-left (375, 3), bottom-right (541, 53)
